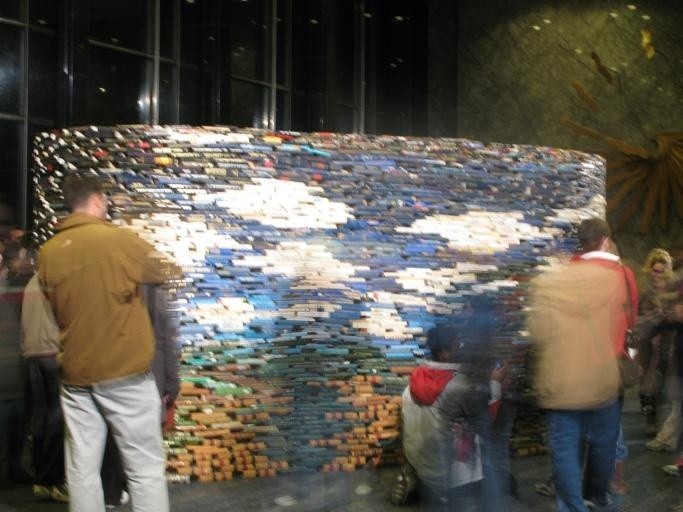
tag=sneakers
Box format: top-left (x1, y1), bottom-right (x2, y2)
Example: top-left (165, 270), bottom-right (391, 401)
top-left (535, 460), bottom-right (630, 512)
top-left (644, 421), bottom-right (683, 477)
top-left (391, 461), bottom-right (418, 507)
top-left (32, 479), bottom-right (129, 509)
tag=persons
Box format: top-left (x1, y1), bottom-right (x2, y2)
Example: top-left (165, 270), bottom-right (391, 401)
top-left (0, 174), bottom-right (171, 512)
top-left (523, 219), bottom-right (683, 512)
top-left (401, 330), bottom-right (519, 512)
